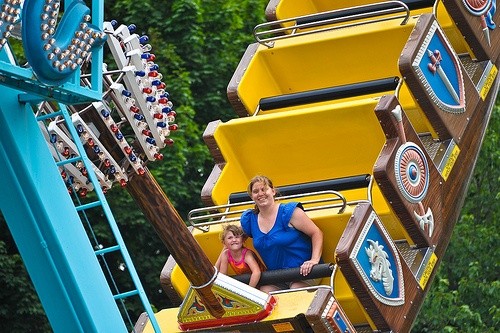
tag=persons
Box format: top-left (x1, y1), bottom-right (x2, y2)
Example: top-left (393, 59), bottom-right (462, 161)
top-left (218, 224), bottom-right (267, 289)
top-left (214, 175), bottom-right (323, 293)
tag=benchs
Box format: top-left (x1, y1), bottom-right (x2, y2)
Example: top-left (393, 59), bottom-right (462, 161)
top-left (133, 0), bottom-right (479, 333)
